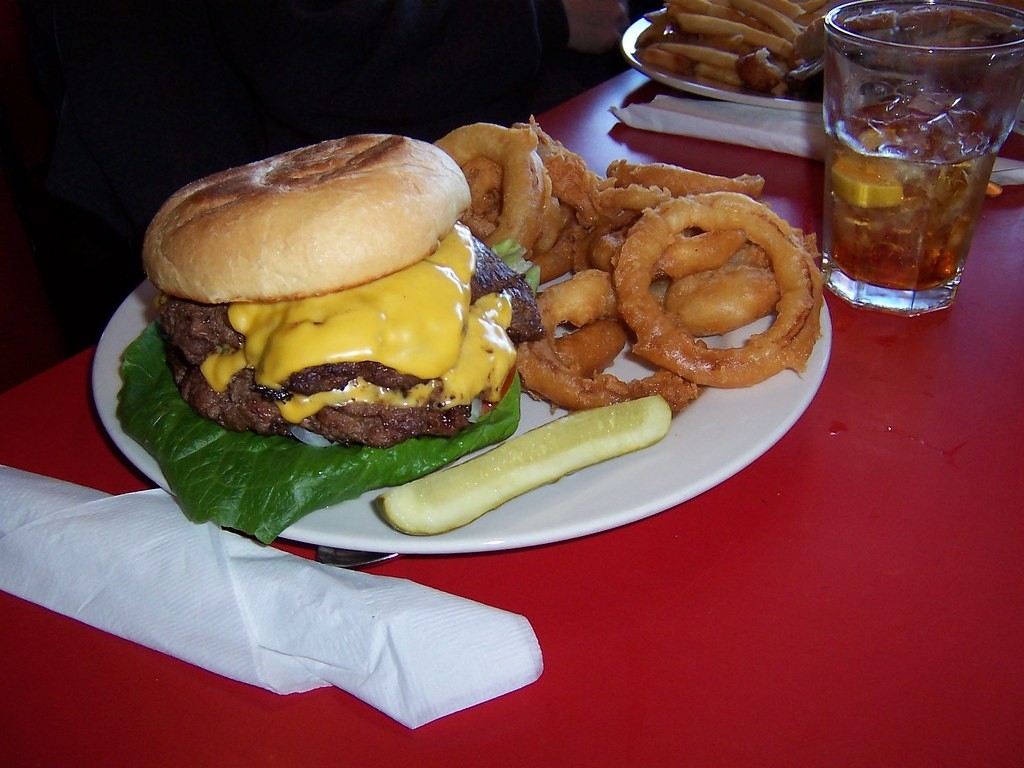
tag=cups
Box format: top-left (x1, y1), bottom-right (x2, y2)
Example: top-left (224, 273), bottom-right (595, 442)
top-left (816, 1), bottom-right (1023, 321)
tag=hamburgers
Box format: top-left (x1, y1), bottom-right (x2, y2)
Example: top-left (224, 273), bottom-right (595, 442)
top-left (116, 133), bottom-right (541, 541)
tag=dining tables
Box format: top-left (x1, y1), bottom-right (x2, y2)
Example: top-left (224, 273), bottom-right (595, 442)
top-left (0, 64), bottom-right (1024, 767)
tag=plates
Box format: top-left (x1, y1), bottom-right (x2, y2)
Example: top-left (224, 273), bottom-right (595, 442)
top-left (91, 275), bottom-right (832, 558)
top-left (618, 10), bottom-right (822, 117)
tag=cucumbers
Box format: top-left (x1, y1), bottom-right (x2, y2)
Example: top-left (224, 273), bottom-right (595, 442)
top-left (380, 395), bottom-right (675, 537)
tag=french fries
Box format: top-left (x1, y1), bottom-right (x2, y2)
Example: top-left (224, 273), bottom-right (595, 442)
top-left (637, 0), bottom-right (830, 99)
